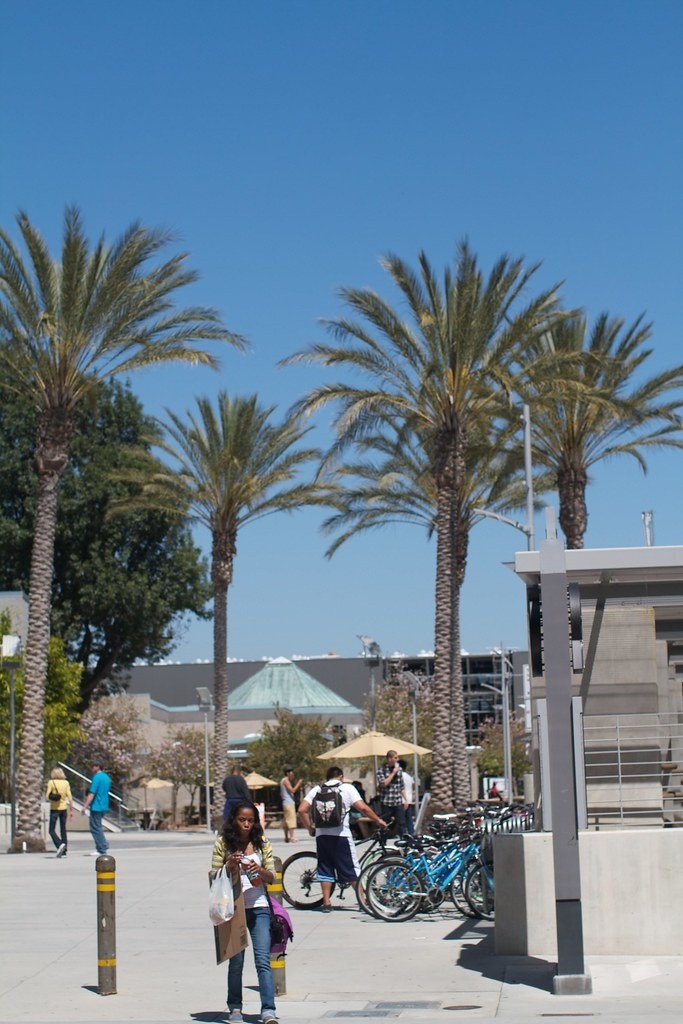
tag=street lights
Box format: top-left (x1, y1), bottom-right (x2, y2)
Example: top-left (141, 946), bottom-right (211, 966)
top-left (358, 634), bottom-right (385, 795)
top-left (403, 670), bottom-right (423, 816)
top-left (0, 634), bottom-right (22, 842)
top-left (195, 686), bottom-right (212, 831)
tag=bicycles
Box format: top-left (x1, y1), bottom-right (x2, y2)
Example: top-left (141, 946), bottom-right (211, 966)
top-left (355, 794), bottom-right (536, 922)
top-left (280, 816), bottom-right (399, 911)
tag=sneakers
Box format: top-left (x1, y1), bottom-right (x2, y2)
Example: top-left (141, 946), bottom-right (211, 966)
top-left (262, 1009), bottom-right (278, 1024)
top-left (228, 1008), bottom-right (244, 1023)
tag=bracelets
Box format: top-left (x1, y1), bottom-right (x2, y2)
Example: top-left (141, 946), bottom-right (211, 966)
top-left (376, 819), bottom-right (383, 825)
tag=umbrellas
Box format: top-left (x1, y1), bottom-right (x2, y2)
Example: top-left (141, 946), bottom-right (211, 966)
top-left (307, 777), bottom-right (353, 785)
top-left (121, 775), bottom-right (174, 809)
top-left (204, 771), bottom-right (278, 802)
top-left (316, 732), bottom-right (432, 797)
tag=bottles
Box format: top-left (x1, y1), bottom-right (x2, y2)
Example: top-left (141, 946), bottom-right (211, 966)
top-left (240, 854), bottom-right (263, 887)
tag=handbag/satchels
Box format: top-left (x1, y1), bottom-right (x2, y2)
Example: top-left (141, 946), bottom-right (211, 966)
top-left (268, 897), bottom-right (293, 953)
top-left (49, 792), bottom-right (61, 801)
top-left (209, 865), bottom-right (234, 927)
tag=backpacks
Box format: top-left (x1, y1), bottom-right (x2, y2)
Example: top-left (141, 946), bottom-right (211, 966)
top-left (311, 784), bottom-right (346, 827)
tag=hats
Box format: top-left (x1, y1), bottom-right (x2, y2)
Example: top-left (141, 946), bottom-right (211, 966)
top-left (284, 765), bottom-right (293, 772)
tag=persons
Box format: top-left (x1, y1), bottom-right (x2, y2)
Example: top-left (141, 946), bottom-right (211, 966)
top-left (349, 750), bottom-right (414, 846)
top-left (279, 765), bottom-right (303, 843)
top-left (46, 767), bottom-right (74, 858)
top-left (298, 767), bottom-right (386, 913)
top-left (222, 764), bottom-right (252, 820)
top-left (208, 801), bottom-right (279, 1024)
top-left (80, 759), bottom-right (112, 856)
top-left (487, 788), bottom-right (499, 799)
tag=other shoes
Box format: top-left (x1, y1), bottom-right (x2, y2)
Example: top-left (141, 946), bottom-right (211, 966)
top-left (322, 903), bottom-right (335, 913)
top-left (90, 851), bottom-right (106, 855)
top-left (56, 843), bottom-right (67, 858)
